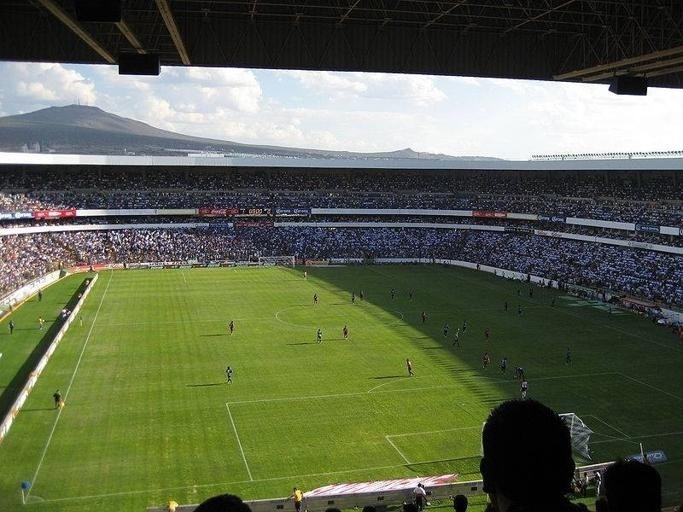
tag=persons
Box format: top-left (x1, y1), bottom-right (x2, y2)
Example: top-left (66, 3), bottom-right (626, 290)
top-left (317, 329), bottom-right (322, 343)
top-left (420, 311), bottom-right (431, 323)
top-left (503, 271), bottom-right (683, 335)
top-left (52, 389), bottom-right (64, 409)
top-left (76, 292), bottom-right (82, 301)
top-left (497, 357), bottom-right (507, 374)
top-left (452, 328), bottom-right (460, 347)
top-left (61, 306), bottom-right (67, 321)
top-left (83, 279), bottom-right (89, 288)
top-left (478, 399), bottom-right (589, 510)
top-left (440, 323), bottom-right (450, 338)
top-left (37, 316), bottom-right (45, 330)
top-left (459, 320), bottom-right (472, 336)
top-left (351, 292), bottom-right (356, 304)
top-left (342, 325), bottom-right (348, 338)
top-left (0, 173), bottom-right (682, 272)
top-left (163, 452), bottom-right (663, 511)
top-left (228, 321), bottom-right (234, 335)
top-left (483, 325), bottom-right (490, 342)
top-left (7, 320), bottom-right (15, 334)
top-left (405, 358), bottom-right (414, 376)
top-left (358, 290), bottom-right (364, 301)
top-left (519, 377), bottom-right (528, 398)
top-left (390, 288), bottom-right (397, 300)
top-left (303, 271), bottom-right (307, 279)
top-left (481, 352), bottom-right (491, 368)
top-left (513, 366), bottom-right (525, 380)
top-left (313, 293), bottom-right (317, 305)
top-left (564, 345), bottom-right (574, 365)
top-left (64, 306), bottom-right (71, 316)
top-left (37, 289), bottom-right (42, 302)
top-left (0, 271), bottom-right (51, 300)
top-left (407, 287), bottom-right (416, 302)
top-left (225, 366), bottom-right (232, 384)
